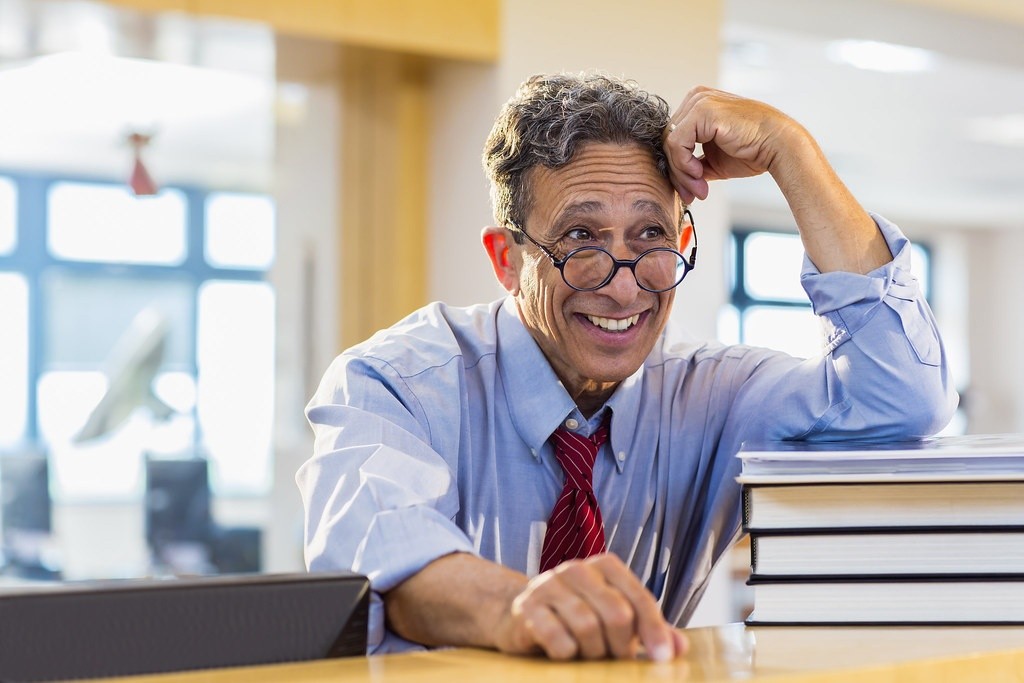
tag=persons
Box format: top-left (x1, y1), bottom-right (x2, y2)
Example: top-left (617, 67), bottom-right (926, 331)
top-left (292, 68), bottom-right (961, 662)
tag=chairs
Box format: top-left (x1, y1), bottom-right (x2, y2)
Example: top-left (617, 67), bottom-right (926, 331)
top-left (144, 459), bottom-right (259, 578)
top-left (1, 569), bottom-right (371, 682)
top-left (0, 447), bottom-right (61, 581)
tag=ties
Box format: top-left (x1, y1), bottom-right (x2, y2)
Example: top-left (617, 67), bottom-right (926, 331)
top-left (535, 407), bottom-right (615, 577)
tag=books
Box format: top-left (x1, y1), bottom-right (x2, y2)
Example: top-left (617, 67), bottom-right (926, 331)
top-left (735, 438), bottom-right (1024, 625)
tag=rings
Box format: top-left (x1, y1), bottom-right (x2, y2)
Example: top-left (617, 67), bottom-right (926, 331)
top-left (670, 123), bottom-right (676, 129)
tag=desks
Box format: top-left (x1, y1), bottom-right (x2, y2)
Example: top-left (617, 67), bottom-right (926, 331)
top-left (57, 623), bottom-right (1024, 683)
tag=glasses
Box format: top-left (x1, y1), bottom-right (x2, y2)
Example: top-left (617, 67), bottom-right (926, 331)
top-left (501, 209), bottom-right (698, 294)
